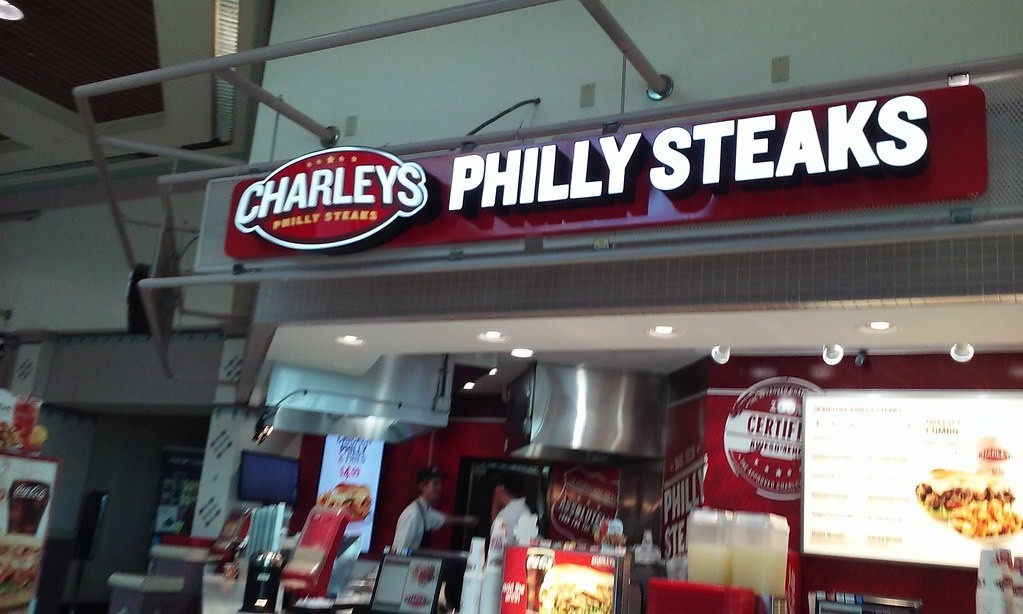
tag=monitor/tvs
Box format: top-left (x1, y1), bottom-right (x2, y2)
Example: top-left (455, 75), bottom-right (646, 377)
top-left (349, 548), bottom-right (466, 613)
top-left (236, 449), bottom-right (301, 506)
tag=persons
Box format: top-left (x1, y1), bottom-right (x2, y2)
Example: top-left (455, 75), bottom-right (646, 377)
top-left (486, 473), bottom-right (539, 565)
top-left (390, 465), bottom-right (478, 554)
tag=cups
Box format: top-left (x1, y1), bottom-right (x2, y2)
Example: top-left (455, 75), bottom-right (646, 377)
top-left (7, 477), bottom-right (50, 538)
top-left (521, 547), bottom-right (554, 614)
top-left (460, 535), bottom-right (504, 614)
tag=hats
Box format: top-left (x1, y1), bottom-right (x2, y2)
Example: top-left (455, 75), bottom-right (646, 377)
top-left (417, 465), bottom-right (448, 485)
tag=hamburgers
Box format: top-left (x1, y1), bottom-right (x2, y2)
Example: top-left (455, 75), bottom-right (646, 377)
top-left (540, 564), bottom-right (614, 614)
top-left (334, 484), bottom-right (371, 521)
top-left (0, 535), bottom-right (40, 607)
top-left (916, 469), bottom-right (1022, 544)
